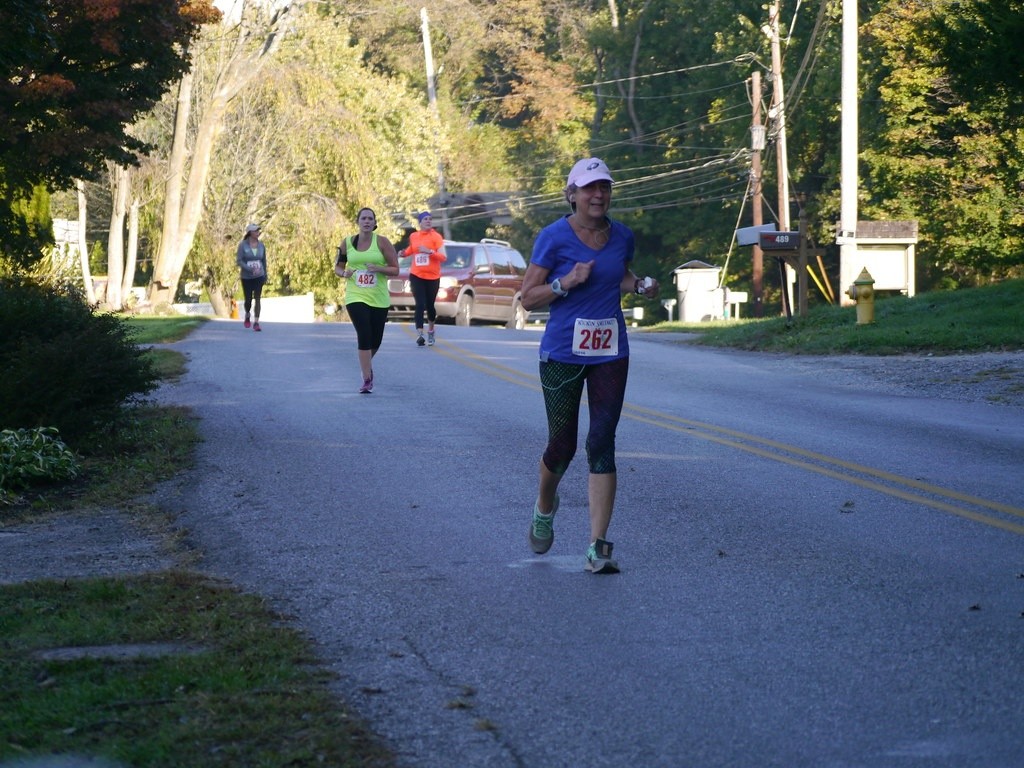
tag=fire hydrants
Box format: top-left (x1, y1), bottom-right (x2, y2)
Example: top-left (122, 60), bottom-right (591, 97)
top-left (845, 267), bottom-right (876, 326)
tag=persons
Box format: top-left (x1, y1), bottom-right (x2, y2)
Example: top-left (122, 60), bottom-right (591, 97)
top-left (236, 223), bottom-right (268, 331)
top-left (335, 208), bottom-right (399, 393)
top-left (521, 158), bottom-right (663, 574)
top-left (398, 211), bottom-right (447, 346)
top-left (450, 254), bottom-right (465, 268)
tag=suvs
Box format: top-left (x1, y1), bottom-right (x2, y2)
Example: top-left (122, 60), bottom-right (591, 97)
top-left (385, 237), bottom-right (533, 331)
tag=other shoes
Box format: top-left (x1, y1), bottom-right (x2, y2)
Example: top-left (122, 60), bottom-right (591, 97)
top-left (244, 315), bottom-right (251, 328)
top-left (252, 322), bottom-right (261, 331)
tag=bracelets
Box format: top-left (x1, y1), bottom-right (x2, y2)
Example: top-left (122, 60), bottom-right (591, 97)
top-left (429, 250), bottom-right (433, 254)
top-left (339, 270), bottom-right (345, 277)
top-left (634, 278), bottom-right (644, 295)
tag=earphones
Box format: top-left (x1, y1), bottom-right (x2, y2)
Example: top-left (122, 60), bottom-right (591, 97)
top-left (569, 194), bottom-right (573, 202)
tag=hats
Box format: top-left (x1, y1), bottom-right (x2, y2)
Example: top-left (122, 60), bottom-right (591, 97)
top-left (567, 158), bottom-right (615, 187)
top-left (245, 224), bottom-right (261, 234)
top-left (418, 212), bottom-right (431, 223)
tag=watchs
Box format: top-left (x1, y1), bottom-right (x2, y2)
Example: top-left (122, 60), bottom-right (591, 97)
top-left (551, 277), bottom-right (568, 298)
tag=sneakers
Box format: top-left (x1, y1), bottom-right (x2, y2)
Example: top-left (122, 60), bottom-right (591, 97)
top-left (427, 331), bottom-right (435, 347)
top-left (415, 332), bottom-right (425, 346)
top-left (528, 493), bottom-right (560, 554)
top-left (584, 538), bottom-right (620, 574)
top-left (360, 379), bottom-right (373, 393)
top-left (362, 367), bottom-right (373, 381)
